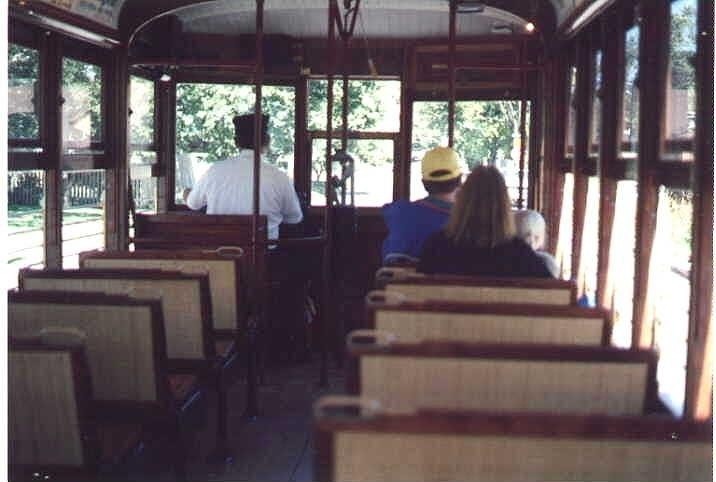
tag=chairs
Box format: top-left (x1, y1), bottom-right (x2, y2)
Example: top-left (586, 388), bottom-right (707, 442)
top-left (8, 209), bottom-right (275, 482)
top-left (312, 263), bottom-right (713, 479)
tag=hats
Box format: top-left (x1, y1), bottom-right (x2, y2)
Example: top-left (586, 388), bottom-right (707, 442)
top-left (422, 148), bottom-right (463, 181)
top-left (233, 113), bottom-right (270, 137)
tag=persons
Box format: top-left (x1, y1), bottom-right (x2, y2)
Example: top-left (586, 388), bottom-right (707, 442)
top-left (377, 146), bottom-right (462, 268)
top-left (182, 115), bottom-right (304, 280)
top-left (415, 165), bottom-right (551, 279)
top-left (515, 211), bottom-right (559, 280)
